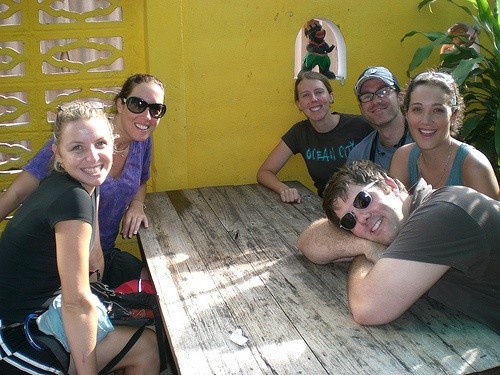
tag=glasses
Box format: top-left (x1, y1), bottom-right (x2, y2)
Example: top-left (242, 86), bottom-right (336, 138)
top-left (123, 96), bottom-right (167, 119)
top-left (339, 179), bottom-right (378, 230)
top-left (359, 85), bottom-right (394, 103)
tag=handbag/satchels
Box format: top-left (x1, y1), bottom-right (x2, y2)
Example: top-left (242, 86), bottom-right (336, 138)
top-left (43, 269), bottom-right (155, 327)
top-left (35, 292), bottom-right (115, 354)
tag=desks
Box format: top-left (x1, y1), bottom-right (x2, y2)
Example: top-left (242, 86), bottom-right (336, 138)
top-left (137, 180), bottom-right (500, 375)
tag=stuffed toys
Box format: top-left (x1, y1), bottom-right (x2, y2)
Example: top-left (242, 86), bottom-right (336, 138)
top-left (299, 19), bottom-right (337, 78)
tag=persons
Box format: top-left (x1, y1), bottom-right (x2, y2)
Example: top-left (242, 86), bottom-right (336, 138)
top-left (255, 70), bottom-right (376, 204)
top-left (0, 100), bottom-right (161, 375)
top-left (1, 72), bottom-right (167, 282)
top-left (297, 161), bottom-right (500, 338)
top-left (387, 70), bottom-right (500, 201)
top-left (343, 65), bottom-right (415, 173)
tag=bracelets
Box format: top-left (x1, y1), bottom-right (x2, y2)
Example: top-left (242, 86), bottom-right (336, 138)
top-left (129, 198), bottom-right (147, 208)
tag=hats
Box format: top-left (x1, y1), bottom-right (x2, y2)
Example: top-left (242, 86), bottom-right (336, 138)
top-left (355, 66), bottom-right (400, 94)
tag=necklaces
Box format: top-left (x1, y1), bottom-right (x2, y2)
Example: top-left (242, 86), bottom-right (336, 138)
top-left (415, 140), bottom-right (453, 186)
